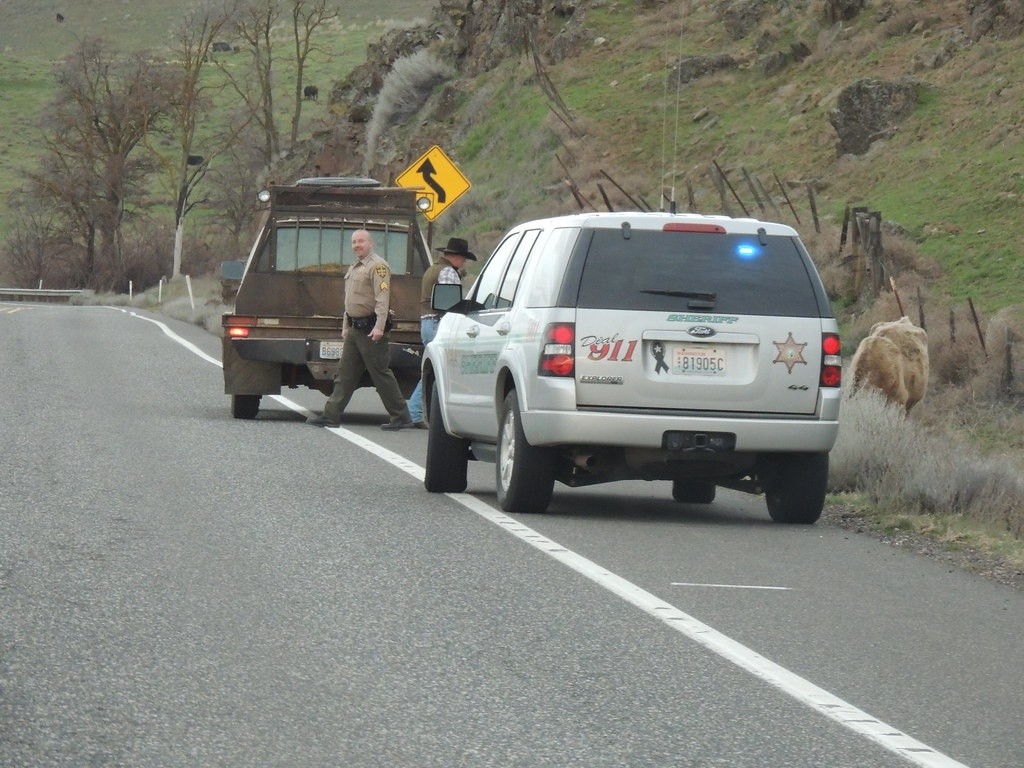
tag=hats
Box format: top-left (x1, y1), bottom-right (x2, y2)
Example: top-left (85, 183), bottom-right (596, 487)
top-left (434, 237), bottom-right (477, 261)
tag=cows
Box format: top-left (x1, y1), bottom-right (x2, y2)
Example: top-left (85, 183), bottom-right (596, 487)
top-left (845, 313), bottom-right (930, 423)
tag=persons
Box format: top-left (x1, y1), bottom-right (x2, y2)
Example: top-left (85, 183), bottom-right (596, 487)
top-left (306, 228), bottom-right (415, 432)
top-left (407, 237), bottom-right (478, 429)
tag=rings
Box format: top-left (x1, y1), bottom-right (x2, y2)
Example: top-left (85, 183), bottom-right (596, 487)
top-left (375, 340), bottom-right (379, 344)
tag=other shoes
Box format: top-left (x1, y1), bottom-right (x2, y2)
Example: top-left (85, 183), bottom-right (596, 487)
top-left (306, 416), bottom-right (339, 428)
top-left (380, 422), bottom-right (414, 431)
top-left (415, 420), bottom-right (428, 429)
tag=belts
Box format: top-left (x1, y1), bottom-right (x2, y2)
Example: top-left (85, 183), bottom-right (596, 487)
top-left (421, 316), bottom-right (438, 320)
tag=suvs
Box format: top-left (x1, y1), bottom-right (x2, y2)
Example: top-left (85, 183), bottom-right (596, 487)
top-left (422, 208), bottom-right (842, 524)
top-left (218, 176), bottom-right (450, 419)
